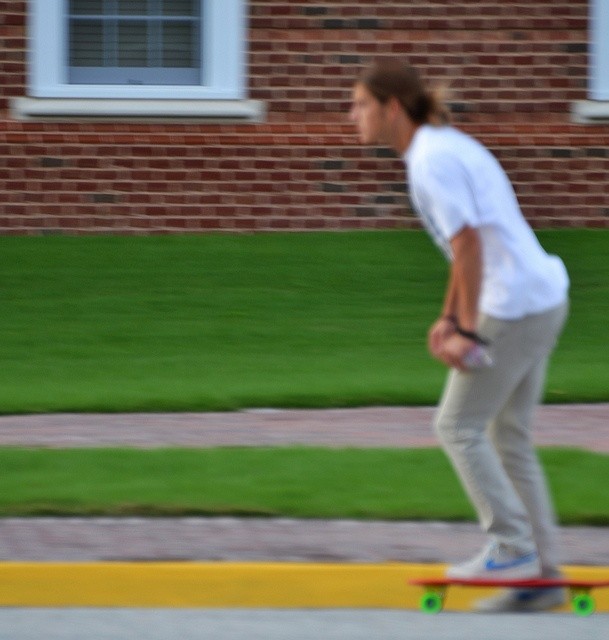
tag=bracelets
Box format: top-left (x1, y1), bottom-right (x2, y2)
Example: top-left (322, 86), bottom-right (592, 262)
top-left (457, 327), bottom-right (494, 344)
top-left (442, 313), bottom-right (457, 323)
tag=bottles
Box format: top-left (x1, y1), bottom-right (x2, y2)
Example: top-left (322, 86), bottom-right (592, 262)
top-left (462, 344), bottom-right (498, 379)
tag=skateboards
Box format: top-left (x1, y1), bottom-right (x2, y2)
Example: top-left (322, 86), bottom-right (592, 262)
top-left (406, 578), bottom-right (609, 616)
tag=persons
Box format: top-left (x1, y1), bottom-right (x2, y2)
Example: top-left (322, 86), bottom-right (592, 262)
top-left (348, 63), bottom-right (569, 609)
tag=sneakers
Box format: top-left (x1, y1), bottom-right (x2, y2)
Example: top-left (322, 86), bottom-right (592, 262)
top-left (449, 542), bottom-right (542, 579)
top-left (475, 586), bottom-right (562, 614)
